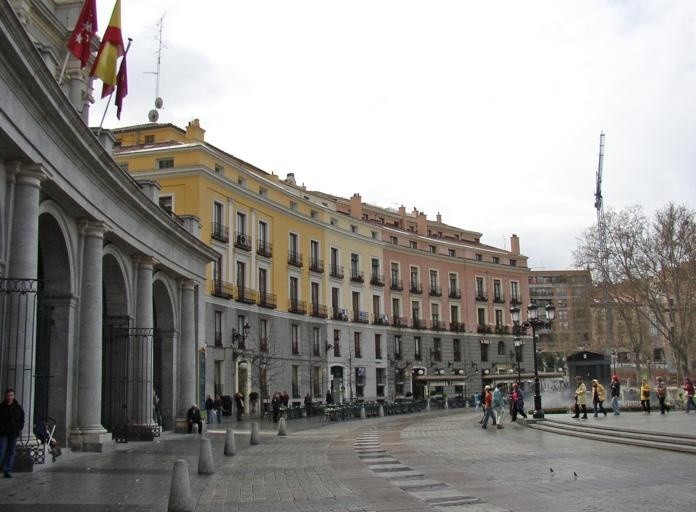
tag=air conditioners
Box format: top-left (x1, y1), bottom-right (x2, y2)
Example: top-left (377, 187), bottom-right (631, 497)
top-left (239, 235), bottom-right (247, 246)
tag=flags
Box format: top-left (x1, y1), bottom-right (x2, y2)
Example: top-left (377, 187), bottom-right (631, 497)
top-left (67, 0), bottom-right (132, 120)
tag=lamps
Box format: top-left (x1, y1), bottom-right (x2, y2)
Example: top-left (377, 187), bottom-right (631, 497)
top-left (232, 322), bottom-right (250, 344)
top-left (325, 335), bottom-right (339, 355)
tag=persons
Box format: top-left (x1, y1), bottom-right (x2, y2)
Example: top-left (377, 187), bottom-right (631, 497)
top-left (609, 375), bottom-right (622, 417)
top-left (0, 388), bottom-right (24, 478)
top-left (638, 378), bottom-right (651, 415)
top-left (572, 375), bottom-right (588, 419)
top-left (188, 389), bottom-right (334, 435)
top-left (681, 377), bottom-right (696, 414)
top-left (590, 378), bottom-right (607, 417)
top-left (473, 382), bottom-right (528, 429)
top-left (655, 375), bottom-right (670, 415)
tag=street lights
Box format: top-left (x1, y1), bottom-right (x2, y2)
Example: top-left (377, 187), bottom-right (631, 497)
top-left (647, 356), bottom-right (653, 384)
top-left (510, 303), bottom-right (557, 419)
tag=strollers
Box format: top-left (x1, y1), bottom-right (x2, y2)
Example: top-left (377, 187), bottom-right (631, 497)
top-left (32, 417), bottom-right (60, 463)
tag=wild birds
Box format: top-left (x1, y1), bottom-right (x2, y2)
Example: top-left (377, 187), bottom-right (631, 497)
top-left (574, 472), bottom-right (577, 477)
top-left (550, 468), bottom-right (554, 473)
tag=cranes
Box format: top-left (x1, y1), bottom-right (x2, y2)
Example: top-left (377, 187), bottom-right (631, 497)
top-left (592, 131), bottom-right (616, 348)
